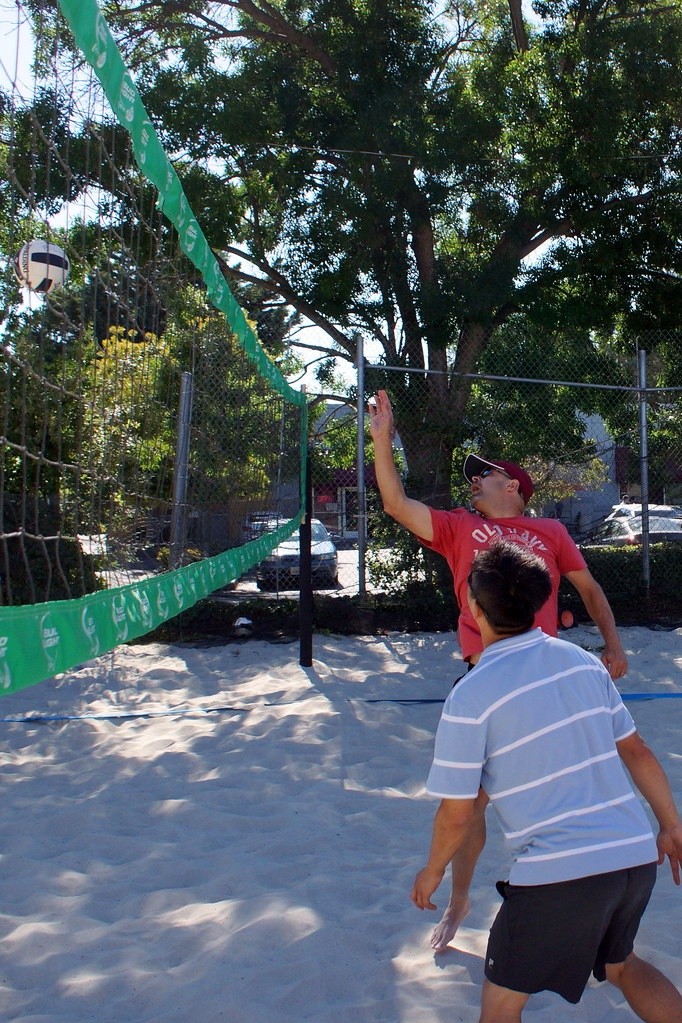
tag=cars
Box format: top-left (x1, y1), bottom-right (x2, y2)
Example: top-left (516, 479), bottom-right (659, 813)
top-left (257, 517), bottom-right (340, 587)
top-left (582, 516), bottom-right (682, 549)
top-left (603, 503), bottom-right (682, 523)
top-left (242, 510), bottom-right (284, 543)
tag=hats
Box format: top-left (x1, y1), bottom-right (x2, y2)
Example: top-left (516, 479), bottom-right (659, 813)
top-left (463, 453), bottom-right (534, 507)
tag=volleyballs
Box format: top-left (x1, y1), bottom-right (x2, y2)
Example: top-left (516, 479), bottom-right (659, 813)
top-left (13, 238), bottom-right (71, 295)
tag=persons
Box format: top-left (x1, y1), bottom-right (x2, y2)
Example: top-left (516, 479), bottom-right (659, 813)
top-left (366, 390), bottom-right (629, 952)
top-left (410, 538), bottom-right (681, 1022)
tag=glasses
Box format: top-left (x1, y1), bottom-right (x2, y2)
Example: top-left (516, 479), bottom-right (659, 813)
top-left (466, 571), bottom-right (489, 619)
top-left (474, 466), bottom-right (512, 480)
